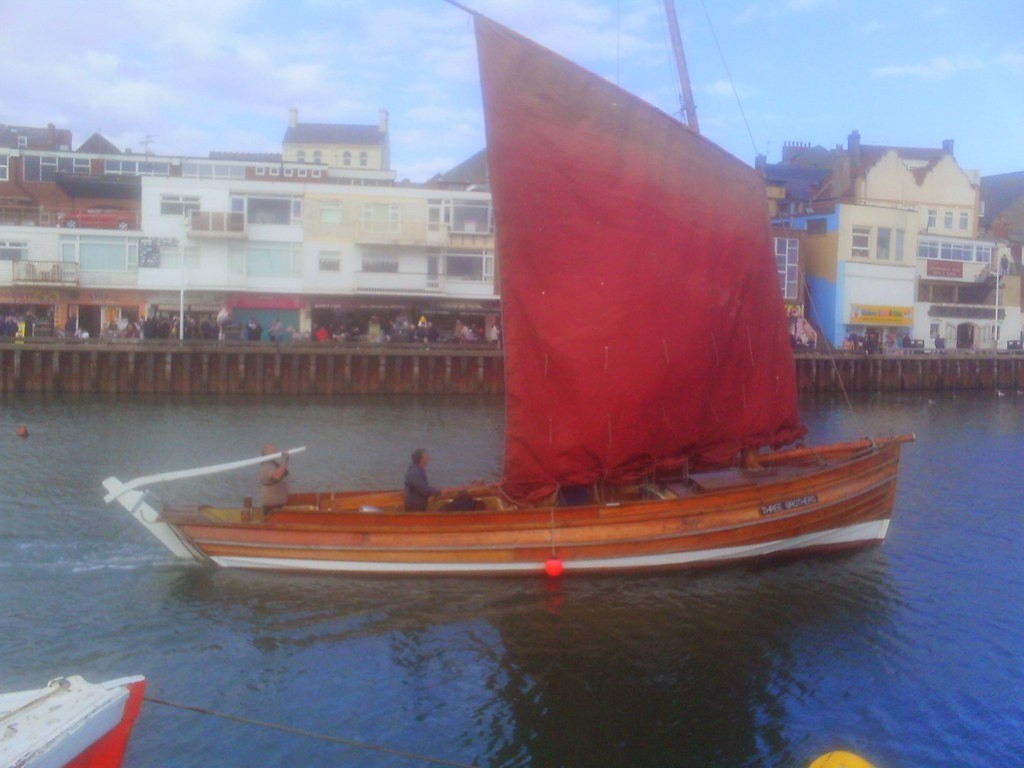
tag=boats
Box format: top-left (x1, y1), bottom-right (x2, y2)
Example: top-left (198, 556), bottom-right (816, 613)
top-left (0, 669), bottom-right (149, 767)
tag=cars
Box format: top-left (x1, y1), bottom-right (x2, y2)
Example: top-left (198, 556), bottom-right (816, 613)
top-left (58, 204), bottom-right (138, 232)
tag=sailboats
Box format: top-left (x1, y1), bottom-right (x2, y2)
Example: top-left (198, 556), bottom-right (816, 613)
top-left (105, 1), bottom-right (922, 579)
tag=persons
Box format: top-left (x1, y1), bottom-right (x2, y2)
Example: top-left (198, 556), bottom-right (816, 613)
top-left (842, 333), bottom-right (912, 355)
top-left (1000, 254), bottom-right (1009, 276)
top-left (258, 442), bottom-right (290, 516)
top-left (402, 447), bottom-right (450, 512)
top-left (934, 334), bottom-right (945, 354)
top-left (0, 307), bottom-right (500, 346)
